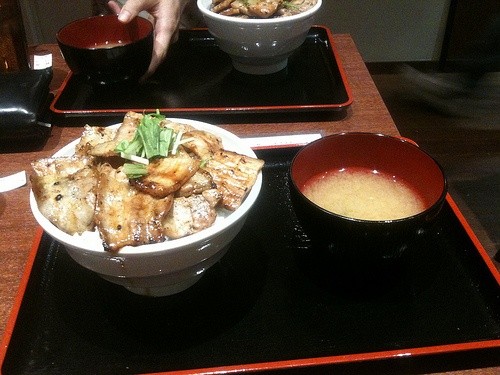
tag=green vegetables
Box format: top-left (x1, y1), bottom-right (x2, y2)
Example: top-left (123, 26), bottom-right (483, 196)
top-left (116, 108), bottom-right (195, 179)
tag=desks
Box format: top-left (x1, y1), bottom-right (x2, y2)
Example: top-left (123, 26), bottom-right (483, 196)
top-left (0, 33), bottom-right (500, 375)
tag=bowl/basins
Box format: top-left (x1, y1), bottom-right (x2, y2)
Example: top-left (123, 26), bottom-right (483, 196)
top-left (197, 0), bottom-right (323, 74)
top-left (288, 132), bottom-right (448, 269)
top-left (55, 14), bottom-right (153, 89)
top-left (29, 118), bottom-right (262, 298)
